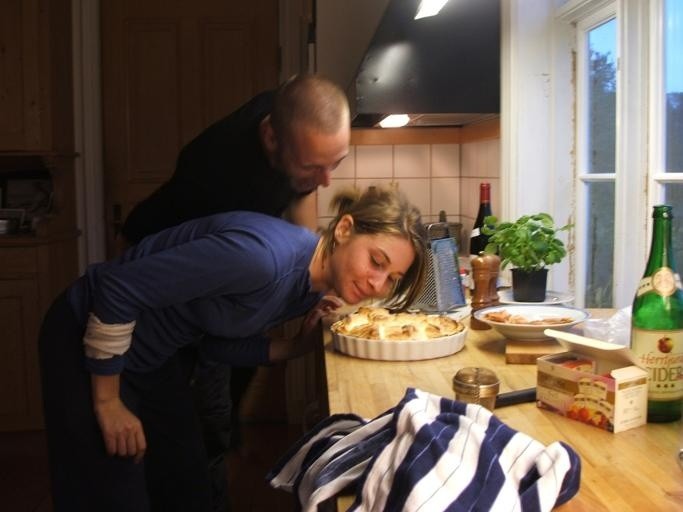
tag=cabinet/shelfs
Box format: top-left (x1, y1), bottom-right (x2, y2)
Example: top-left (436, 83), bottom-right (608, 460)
top-left (1, 1), bottom-right (317, 434)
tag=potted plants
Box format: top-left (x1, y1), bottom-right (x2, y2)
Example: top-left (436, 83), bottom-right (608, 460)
top-left (481, 212), bottom-right (575, 302)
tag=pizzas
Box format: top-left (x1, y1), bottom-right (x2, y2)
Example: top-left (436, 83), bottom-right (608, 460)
top-left (331, 307), bottom-right (465, 342)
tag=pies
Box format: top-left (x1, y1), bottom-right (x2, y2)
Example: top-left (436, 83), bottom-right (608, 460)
top-left (483, 310), bottom-right (575, 324)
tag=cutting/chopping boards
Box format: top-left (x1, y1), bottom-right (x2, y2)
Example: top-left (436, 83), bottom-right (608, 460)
top-left (504, 329), bottom-right (585, 364)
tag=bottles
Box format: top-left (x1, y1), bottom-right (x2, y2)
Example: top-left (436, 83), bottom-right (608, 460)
top-left (468, 182), bottom-right (499, 295)
top-left (627, 205), bottom-right (683, 424)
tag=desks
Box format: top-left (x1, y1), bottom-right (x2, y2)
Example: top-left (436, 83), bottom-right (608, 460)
top-left (322, 257), bottom-right (682, 512)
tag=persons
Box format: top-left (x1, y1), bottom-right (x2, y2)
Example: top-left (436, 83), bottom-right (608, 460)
top-left (120, 70), bottom-right (351, 454)
top-left (38, 183), bottom-right (428, 511)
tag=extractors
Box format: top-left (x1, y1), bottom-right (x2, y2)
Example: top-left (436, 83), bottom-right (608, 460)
top-left (344, 0), bottom-right (502, 130)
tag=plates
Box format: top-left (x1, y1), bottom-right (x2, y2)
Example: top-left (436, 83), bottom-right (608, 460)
top-left (472, 306), bottom-right (593, 342)
top-left (496, 288), bottom-right (577, 306)
top-left (329, 314), bottom-right (469, 363)
